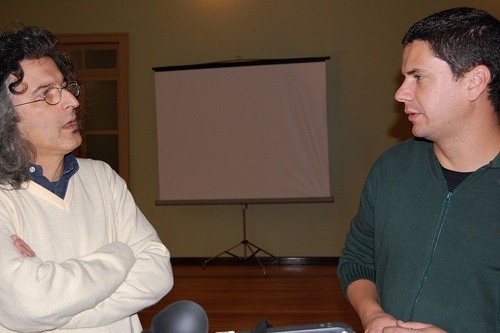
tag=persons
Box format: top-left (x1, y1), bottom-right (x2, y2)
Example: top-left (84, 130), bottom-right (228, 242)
top-left (336, 7), bottom-right (500, 333)
top-left (0, 22), bottom-right (174, 333)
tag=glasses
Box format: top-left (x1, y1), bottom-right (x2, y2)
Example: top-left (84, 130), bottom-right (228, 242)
top-left (11, 80), bottom-right (80, 107)
